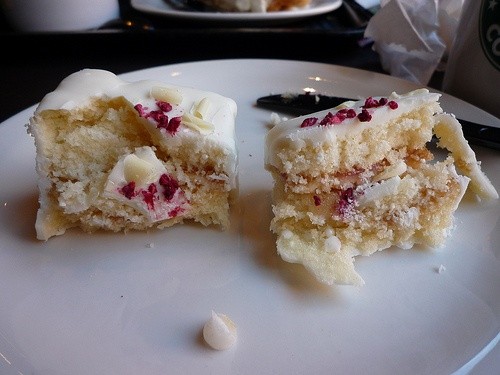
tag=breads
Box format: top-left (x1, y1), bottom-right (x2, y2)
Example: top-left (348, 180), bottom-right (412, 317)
top-left (23, 68), bottom-right (239, 242)
top-left (262, 86), bottom-right (500, 289)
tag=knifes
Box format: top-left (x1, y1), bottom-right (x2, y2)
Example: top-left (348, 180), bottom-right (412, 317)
top-left (258, 93), bottom-right (500, 155)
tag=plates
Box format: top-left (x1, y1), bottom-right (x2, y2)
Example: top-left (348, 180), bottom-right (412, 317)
top-left (130, 0), bottom-right (343, 19)
top-left (0, 57), bottom-right (499, 375)
